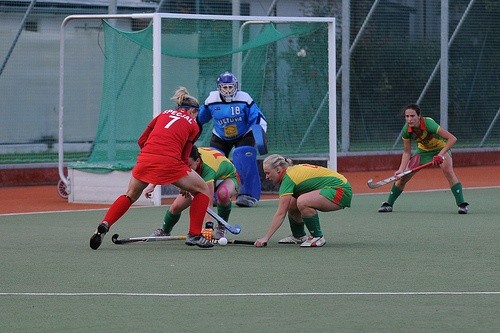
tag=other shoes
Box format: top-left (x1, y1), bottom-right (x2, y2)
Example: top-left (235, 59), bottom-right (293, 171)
top-left (236, 198), bottom-right (249, 206)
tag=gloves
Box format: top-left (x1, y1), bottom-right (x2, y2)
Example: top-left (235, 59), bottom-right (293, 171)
top-left (433, 155), bottom-right (444, 165)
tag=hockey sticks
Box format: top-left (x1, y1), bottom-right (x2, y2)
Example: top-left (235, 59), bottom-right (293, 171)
top-left (111, 233), bottom-right (187, 245)
top-left (211, 237), bottom-right (267, 248)
top-left (171, 183), bottom-right (241, 235)
top-left (367, 156), bottom-right (447, 190)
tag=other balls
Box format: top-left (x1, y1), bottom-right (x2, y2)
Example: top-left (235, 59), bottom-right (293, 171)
top-left (217, 237), bottom-right (228, 246)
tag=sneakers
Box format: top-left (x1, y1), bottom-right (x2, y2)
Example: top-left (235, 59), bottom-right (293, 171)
top-left (300, 235), bottom-right (326, 246)
top-left (378, 201), bottom-right (393, 211)
top-left (458, 201), bottom-right (469, 213)
top-left (215, 227), bottom-right (227, 238)
top-left (278, 233), bottom-right (307, 244)
top-left (90, 223), bottom-right (108, 250)
top-left (185, 232), bottom-right (214, 247)
top-left (148, 227), bottom-right (171, 236)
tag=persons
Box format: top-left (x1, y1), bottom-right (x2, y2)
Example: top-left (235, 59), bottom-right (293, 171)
top-left (144, 145), bottom-right (240, 244)
top-left (89, 86), bottom-right (214, 250)
top-left (197, 71), bottom-right (268, 208)
top-left (378, 103), bottom-right (469, 214)
top-left (254, 154), bottom-right (352, 246)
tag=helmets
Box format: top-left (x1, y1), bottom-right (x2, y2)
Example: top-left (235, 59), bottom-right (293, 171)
top-left (216, 71), bottom-right (238, 102)
